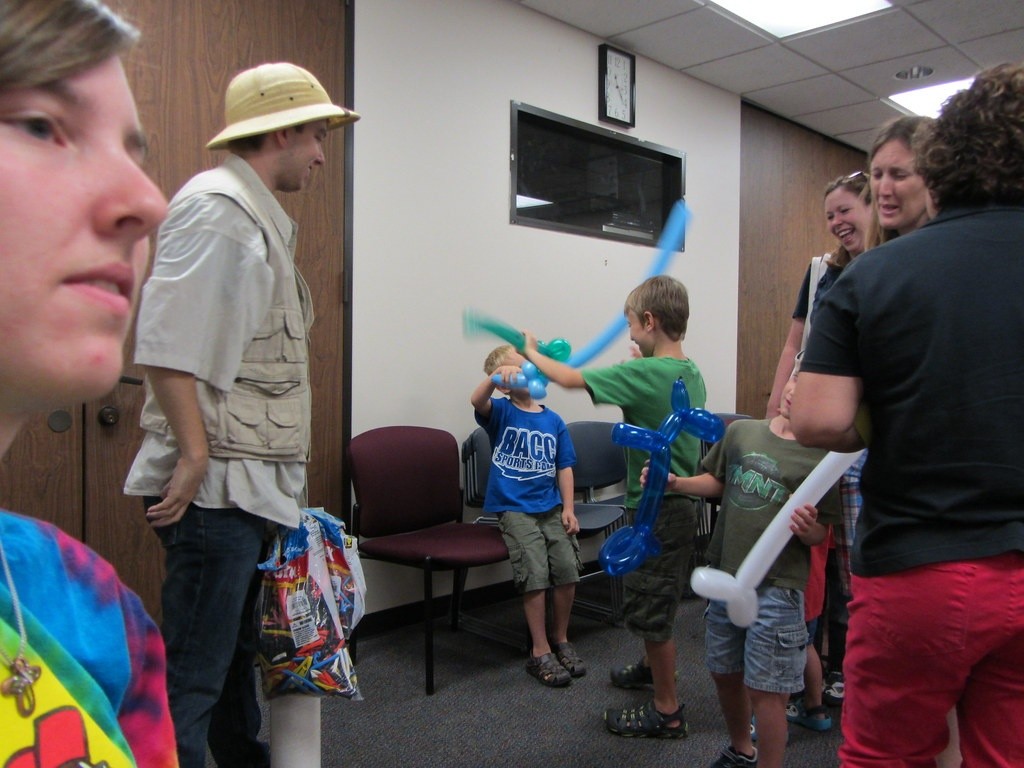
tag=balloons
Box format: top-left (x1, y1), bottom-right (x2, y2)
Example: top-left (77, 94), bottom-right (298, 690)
top-left (690, 448), bottom-right (865, 627)
top-left (598, 379), bottom-right (725, 576)
top-left (465, 311), bottom-right (572, 375)
top-left (492, 200), bottom-right (691, 399)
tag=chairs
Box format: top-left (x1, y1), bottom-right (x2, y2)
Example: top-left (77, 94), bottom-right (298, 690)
top-left (341, 411), bottom-right (759, 694)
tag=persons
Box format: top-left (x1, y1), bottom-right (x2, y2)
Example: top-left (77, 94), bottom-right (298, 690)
top-left (123, 63), bottom-right (363, 768)
top-left (640, 348), bottom-right (845, 768)
top-left (0, 0), bottom-right (180, 768)
top-left (790, 62), bottom-right (1024, 768)
top-left (867, 116), bottom-right (935, 250)
top-left (522, 275), bottom-right (707, 739)
top-left (749, 528), bottom-right (832, 743)
top-left (765, 171), bottom-right (874, 707)
top-left (471, 344), bottom-right (588, 687)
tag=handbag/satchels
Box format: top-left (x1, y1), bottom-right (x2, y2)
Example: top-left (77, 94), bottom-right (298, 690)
top-left (250, 509), bottom-right (364, 704)
top-left (794, 250), bottom-right (832, 373)
top-left (306, 501), bottom-right (371, 643)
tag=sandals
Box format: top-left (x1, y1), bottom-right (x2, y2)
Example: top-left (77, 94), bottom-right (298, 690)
top-left (602, 700), bottom-right (690, 739)
top-left (784, 699), bottom-right (832, 731)
top-left (554, 640), bottom-right (587, 678)
top-left (748, 715), bottom-right (757, 740)
top-left (610, 657), bottom-right (679, 690)
top-left (525, 649), bottom-right (574, 687)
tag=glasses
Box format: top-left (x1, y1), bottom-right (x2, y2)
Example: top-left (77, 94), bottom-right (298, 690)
top-left (847, 169), bottom-right (868, 184)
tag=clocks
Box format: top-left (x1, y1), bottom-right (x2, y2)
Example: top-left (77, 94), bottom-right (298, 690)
top-left (596, 44), bottom-right (635, 127)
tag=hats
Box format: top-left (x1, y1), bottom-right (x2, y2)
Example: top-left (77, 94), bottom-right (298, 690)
top-left (204, 62), bottom-right (361, 150)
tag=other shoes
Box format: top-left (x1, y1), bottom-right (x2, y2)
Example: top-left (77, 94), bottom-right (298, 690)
top-left (819, 672), bottom-right (845, 703)
top-left (707, 745), bottom-right (759, 768)
